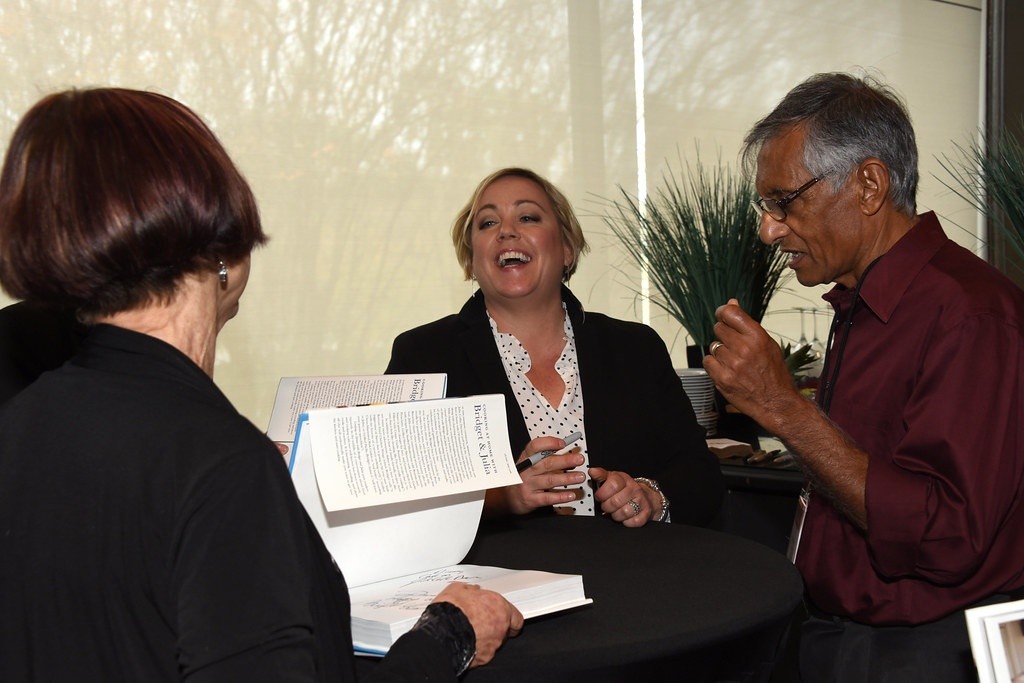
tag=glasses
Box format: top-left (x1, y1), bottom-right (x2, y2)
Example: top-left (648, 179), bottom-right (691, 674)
top-left (750, 167), bottom-right (836, 221)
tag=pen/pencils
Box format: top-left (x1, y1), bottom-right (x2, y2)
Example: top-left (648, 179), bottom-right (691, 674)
top-left (516, 431), bottom-right (582, 473)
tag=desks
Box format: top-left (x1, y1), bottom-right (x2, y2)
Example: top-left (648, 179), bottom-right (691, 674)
top-left (352, 518), bottom-right (808, 683)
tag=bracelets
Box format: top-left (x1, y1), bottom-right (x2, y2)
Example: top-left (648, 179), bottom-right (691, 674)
top-left (634, 477), bottom-right (672, 523)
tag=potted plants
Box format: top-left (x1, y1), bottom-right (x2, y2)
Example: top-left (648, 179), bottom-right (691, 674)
top-left (574, 133), bottom-right (829, 369)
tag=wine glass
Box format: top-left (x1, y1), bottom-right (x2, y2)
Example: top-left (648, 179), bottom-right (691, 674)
top-left (791, 307), bottom-right (825, 378)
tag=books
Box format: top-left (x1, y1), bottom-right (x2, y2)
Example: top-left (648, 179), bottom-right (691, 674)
top-left (263, 370), bottom-right (595, 662)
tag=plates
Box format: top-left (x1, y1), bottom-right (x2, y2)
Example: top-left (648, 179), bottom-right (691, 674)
top-left (674, 368), bottom-right (719, 436)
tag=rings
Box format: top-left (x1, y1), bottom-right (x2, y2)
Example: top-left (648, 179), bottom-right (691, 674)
top-left (711, 341), bottom-right (724, 358)
top-left (626, 499), bottom-right (641, 515)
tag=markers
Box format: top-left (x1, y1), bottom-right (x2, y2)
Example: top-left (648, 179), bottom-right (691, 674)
top-left (754, 449), bottom-right (781, 462)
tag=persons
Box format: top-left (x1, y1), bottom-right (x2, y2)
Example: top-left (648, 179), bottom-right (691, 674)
top-left (0, 86), bottom-right (525, 683)
top-left (693, 70), bottom-right (1024, 682)
top-left (380, 165), bottom-right (723, 527)
